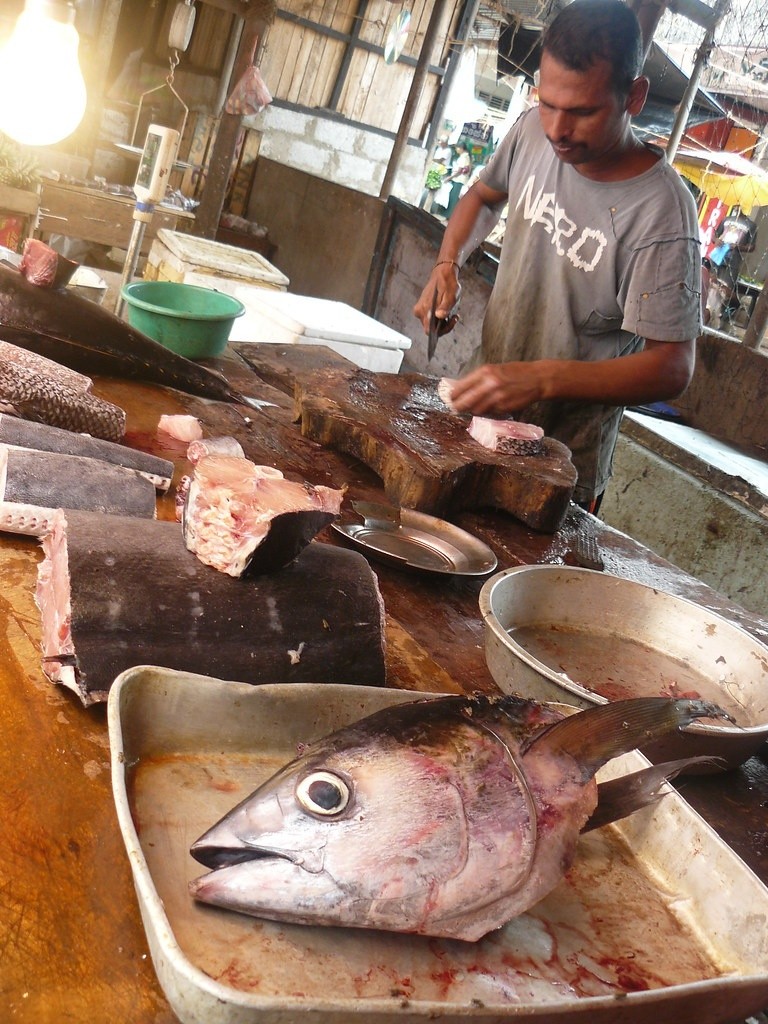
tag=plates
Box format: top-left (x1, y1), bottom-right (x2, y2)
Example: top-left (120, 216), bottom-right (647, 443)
top-left (330, 499), bottom-right (498, 582)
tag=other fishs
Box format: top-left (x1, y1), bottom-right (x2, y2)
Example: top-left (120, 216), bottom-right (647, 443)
top-left (0, 235), bottom-right (725, 943)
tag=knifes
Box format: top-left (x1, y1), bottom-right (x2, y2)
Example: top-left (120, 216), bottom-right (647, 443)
top-left (428, 280), bottom-right (447, 366)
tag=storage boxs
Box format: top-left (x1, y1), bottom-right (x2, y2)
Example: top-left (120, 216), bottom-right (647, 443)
top-left (182, 272), bottom-right (412, 374)
top-left (143, 228), bottom-right (290, 292)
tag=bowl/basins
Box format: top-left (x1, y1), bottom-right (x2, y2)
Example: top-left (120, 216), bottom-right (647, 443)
top-left (120, 281), bottom-right (246, 361)
top-left (479, 565), bottom-right (768, 776)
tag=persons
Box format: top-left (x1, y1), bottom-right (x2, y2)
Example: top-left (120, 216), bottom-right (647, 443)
top-left (421, 135), bottom-right (493, 220)
top-left (415, 0), bottom-right (703, 514)
top-left (702, 206), bottom-right (757, 331)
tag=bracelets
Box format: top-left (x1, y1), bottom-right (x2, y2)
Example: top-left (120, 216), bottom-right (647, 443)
top-left (433, 261), bottom-right (462, 272)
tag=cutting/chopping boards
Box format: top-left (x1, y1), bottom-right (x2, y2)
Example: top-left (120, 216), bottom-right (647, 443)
top-left (292, 366), bottom-right (579, 533)
